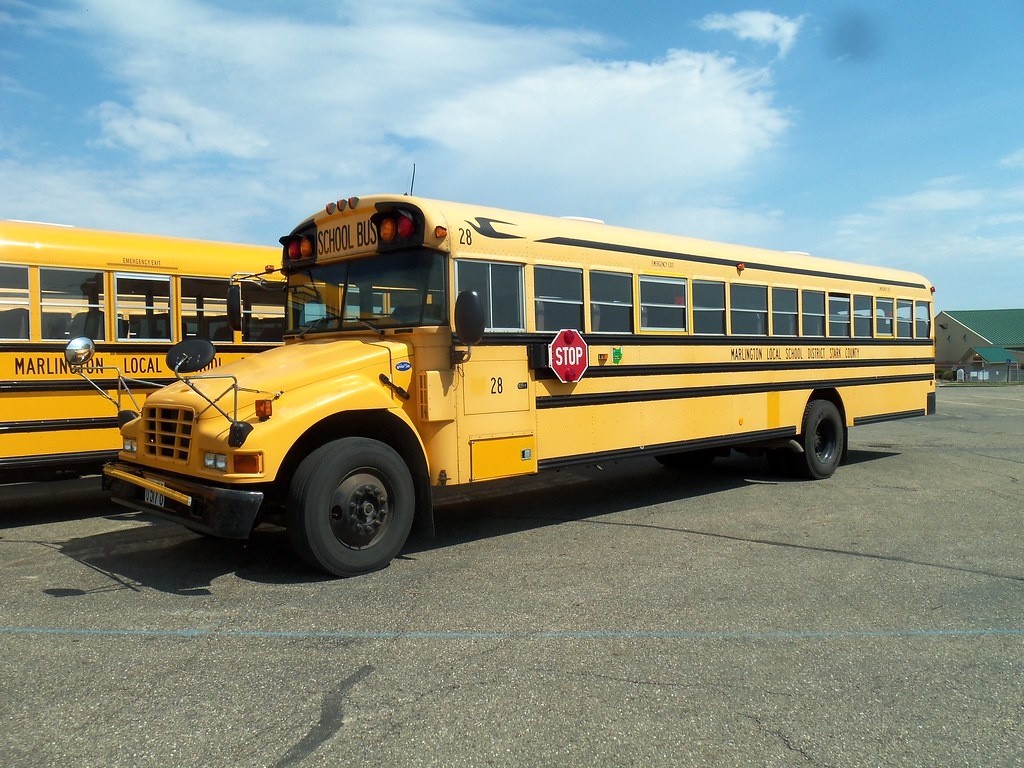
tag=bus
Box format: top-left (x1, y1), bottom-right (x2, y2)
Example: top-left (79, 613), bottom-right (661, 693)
top-left (61, 191), bottom-right (939, 577)
top-left (0, 221), bottom-right (435, 492)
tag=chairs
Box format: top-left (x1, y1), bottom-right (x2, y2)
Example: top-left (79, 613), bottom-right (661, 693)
top-left (0, 308), bottom-right (287, 342)
top-left (494, 290), bottom-right (928, 339)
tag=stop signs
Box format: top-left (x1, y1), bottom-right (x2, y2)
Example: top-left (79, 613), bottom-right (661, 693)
top-left (548, 329), bottom-right (590, 384)
